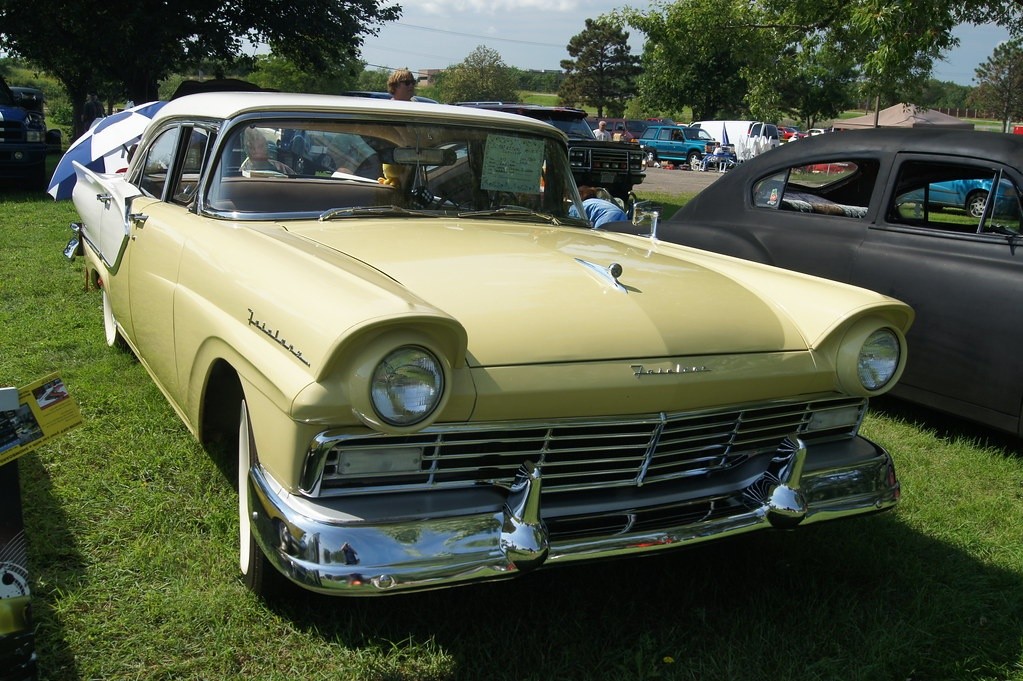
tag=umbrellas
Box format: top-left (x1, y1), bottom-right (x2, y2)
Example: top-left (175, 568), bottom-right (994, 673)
top-left (723, 123), bottom-right (729, 145)
top-left (48, 101), bottom-right (169, 202)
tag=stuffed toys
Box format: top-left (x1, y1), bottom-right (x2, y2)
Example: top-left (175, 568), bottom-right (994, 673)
top-left (376, 163), bottom-right (405, 188)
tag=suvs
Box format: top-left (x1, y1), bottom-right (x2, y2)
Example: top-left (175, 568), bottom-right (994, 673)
top-left (0, 85), bottom-right (62, 190)
top-left (638, 125), bottom-right (736, 171)
top-left (453, 101), bottom-right (648, 203)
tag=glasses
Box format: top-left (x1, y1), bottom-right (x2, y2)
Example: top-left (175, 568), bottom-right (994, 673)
top-left (395, 80), bottom-right (417, 86)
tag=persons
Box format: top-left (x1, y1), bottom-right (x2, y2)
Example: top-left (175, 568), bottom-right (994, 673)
top-left (81, 94), bottom-right (98, 136)
top-left (387, 67), bottom-right (419, 102)
top-left (116, 145), bottom-right (138, 174)
top-left (592, 120), bottom-right (613, 142)
top-left (804, 129), bottom-right (812, 138)
top-left (125, 96), bottom-right (134, 109)
top-left (700, 142), bottom-right (725, 171)
top-left (91, 93), bottom-right (106, 117)
top-left (787, 131), bottom-right (800, 144)
top-left (236, 125), bottom-right (296, 178)
top-left (45, 384), bottom-right (66, 401)
top-left (613, 124), bottom-right (637, 142)
top-left (570, 185), bottom-right (628, 227)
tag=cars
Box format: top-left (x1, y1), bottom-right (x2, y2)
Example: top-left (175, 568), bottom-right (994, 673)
top-left (597, 126), bottom-right (1023, 445)
top-left (778, 127), bottom-right (806, 141)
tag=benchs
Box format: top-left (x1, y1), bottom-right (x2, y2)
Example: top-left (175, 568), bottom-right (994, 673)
top-left (789, 202), bottom-right (898, 226)
top-left (140, 178), bottom-right (417, 219)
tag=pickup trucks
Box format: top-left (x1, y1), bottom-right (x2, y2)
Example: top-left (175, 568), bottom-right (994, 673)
top-left (69, 93), bottom-right (914, 599)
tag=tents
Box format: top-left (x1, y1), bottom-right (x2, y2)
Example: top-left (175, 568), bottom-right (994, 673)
top-left (825, 102), bottom-right (975, 179)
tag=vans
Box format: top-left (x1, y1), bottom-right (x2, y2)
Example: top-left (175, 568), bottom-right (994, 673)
top-left (687, 121), bottom-right (780, 162)
top-left (584, 116), bottom-right (648, 140)
top-left (647, 118), bottom-right (678, 127)
top-left (806, 129), bottom-right (829, 137)
